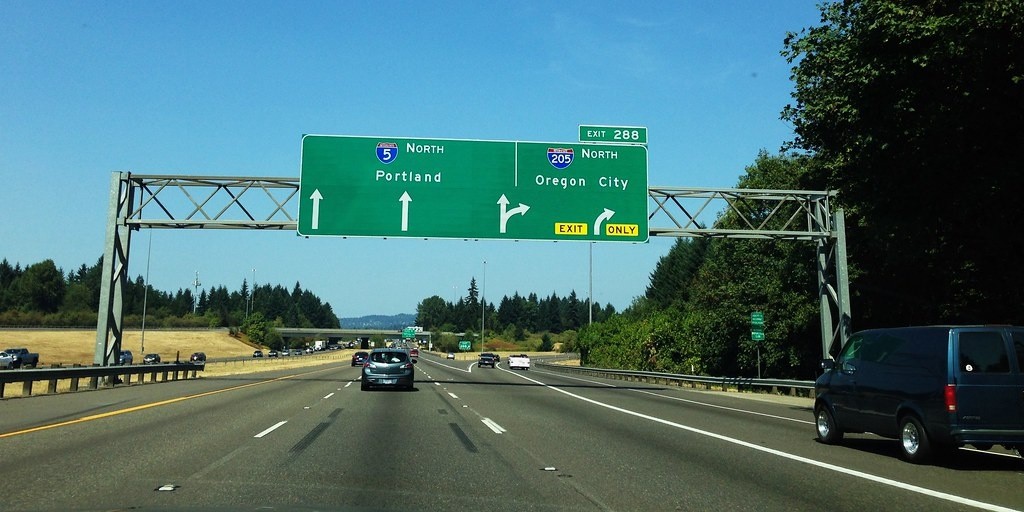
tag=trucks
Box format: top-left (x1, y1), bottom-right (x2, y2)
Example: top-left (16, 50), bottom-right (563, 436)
top-left (315, 341), bottom-right (325, 351)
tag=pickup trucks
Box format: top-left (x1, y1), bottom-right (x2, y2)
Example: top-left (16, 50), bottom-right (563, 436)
top-left (6, 347), bottom-right (39, 369)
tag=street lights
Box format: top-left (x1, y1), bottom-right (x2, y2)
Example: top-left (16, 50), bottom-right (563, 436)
top-left (480, 256), bottom-right (489, 351)
top-left (250, 266), bottom-right (258, 312)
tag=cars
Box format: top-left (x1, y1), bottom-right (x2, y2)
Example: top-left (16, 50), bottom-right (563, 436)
top-left (295, 350), bottom-right (302, 355)
top-left (478, 352), bottom-right (494, 368)
top-left (508, 353), bottom-right (531, 370)
top-left (385, 338), bottom-right (418, 348)
top-left (190, 352), bottom-right (206, 362)
top-left (252, 350), bottom-right (263, 358)
top-left (0, 351), bottom-right (14, 369)
top-left (328, 338), bottom-right (362, 350)
top-left (494, 354), bottom-right (500, 362)
top-left (281, 350), bottom-right (290, 356)
top-left (410, 348), bottom-right (419, 357)
top-left (360, 348), bottom-right (417, 392)
top-left (305, 348), bottom-right (313, 354)
top-left (447, 352), bottom-right (455, 360)
top-left (143, 353), bottom-right (160, 365)
top-left (351, 351), bottom-right (369, 367)
top-left (119, 349), bottom-right (133, 365)
top-left (267, 350), bottom-right (279, 358)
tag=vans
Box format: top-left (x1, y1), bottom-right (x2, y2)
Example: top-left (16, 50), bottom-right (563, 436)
top-left (812, 323), bottom-right (1023, 464)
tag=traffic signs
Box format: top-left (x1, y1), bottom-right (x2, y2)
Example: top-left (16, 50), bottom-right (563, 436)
top-left (298, 134), bottom-right (649, 244)
